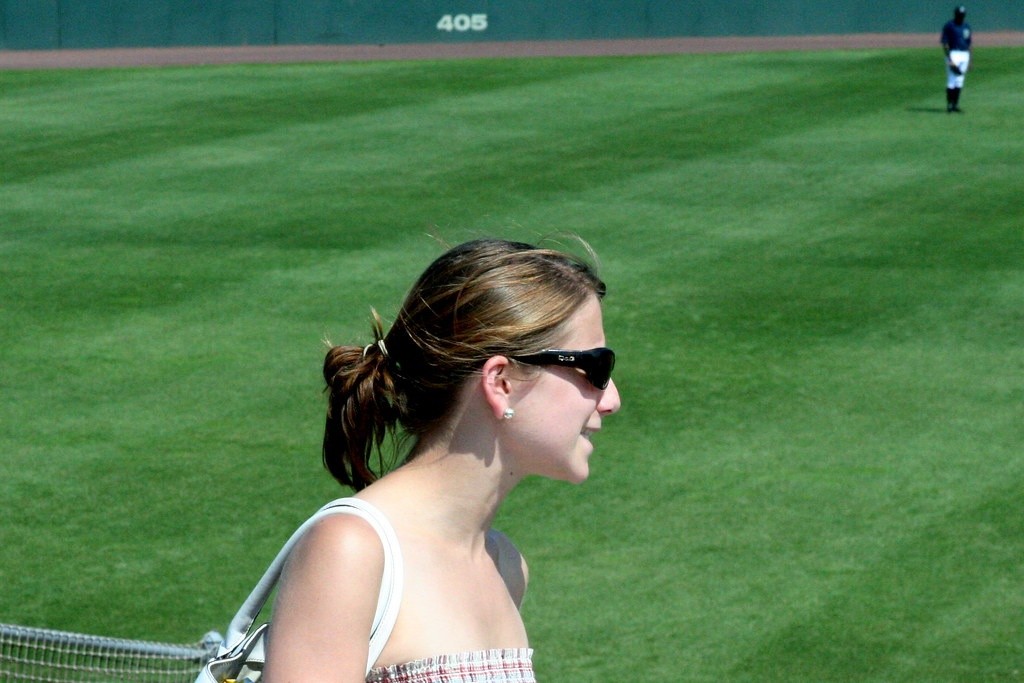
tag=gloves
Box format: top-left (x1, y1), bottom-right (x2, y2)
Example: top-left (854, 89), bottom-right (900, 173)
top-left (950, 64), bottom-right (961, 75)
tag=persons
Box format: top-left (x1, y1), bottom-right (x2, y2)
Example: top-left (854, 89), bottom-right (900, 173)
top-left (258, 239), bottom-right (621, 683)
top-left (941, 5), bottom-right (972, 113)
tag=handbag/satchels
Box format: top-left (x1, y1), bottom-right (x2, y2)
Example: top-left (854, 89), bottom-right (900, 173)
top-left (196, 495), bottom-right (405, 683)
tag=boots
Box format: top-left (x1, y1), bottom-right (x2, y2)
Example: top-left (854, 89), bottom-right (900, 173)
top-left (946, 87), bottom-right (961, 113)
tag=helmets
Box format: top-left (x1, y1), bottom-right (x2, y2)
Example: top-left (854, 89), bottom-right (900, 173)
top-left (955, 6), bottom-right (965, 18)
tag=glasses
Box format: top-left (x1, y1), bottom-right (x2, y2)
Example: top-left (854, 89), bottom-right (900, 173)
top-left (513, 348), bottom-right (616, 391)
